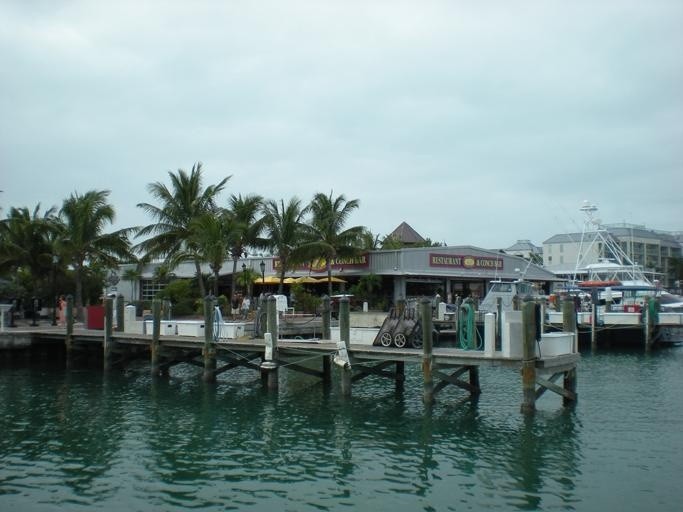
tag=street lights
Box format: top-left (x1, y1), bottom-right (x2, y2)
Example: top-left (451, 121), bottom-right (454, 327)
top-left (240, 261), bottom-right (249, 296)
top-left (258, 261), bottom-right (266, 292)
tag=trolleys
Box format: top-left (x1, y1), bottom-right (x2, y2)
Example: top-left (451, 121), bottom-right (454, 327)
top-left (371, 301), bottom-right (440, 348)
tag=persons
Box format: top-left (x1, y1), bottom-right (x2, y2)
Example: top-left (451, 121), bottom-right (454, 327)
top-left (435, 291), bottom-right (440, 297)
top-left (230, 290), bottom-right (251, 321)
top-left (57, 295), bottom-right (67, 327)
top-left (455, 294), bottom-right (459, 304)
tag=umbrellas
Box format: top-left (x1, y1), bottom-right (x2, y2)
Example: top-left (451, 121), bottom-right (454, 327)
top-left (255, 275), bottom-right (348, 296)
